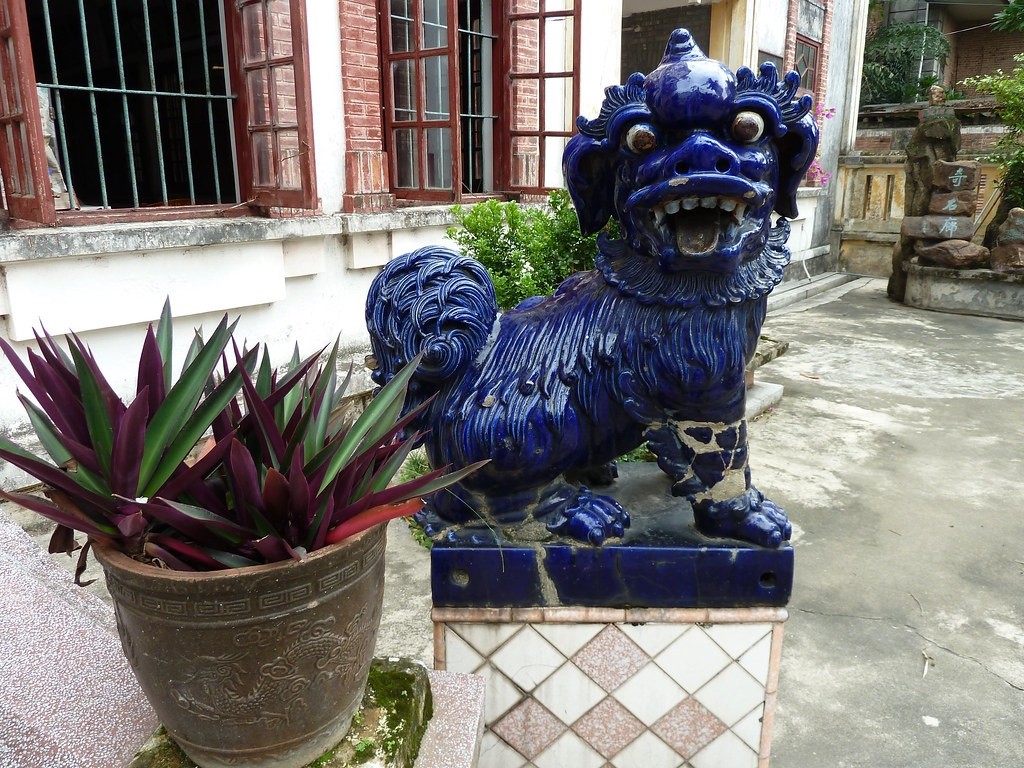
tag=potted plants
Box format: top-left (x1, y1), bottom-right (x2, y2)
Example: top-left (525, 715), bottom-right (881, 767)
top-left (0, 297), bottom-right (491, 768)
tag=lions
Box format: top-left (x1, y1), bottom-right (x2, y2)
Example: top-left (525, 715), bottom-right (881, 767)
top-left (364, 28), bottom-right (816, 549)
